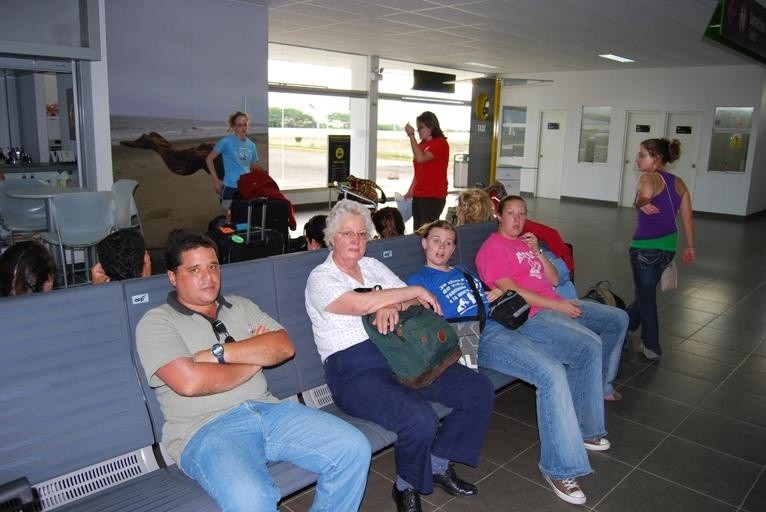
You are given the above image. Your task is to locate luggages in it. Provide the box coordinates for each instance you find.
[232,190,289,253]
[208,197,284,263]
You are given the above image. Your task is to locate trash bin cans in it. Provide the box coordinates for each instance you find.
[453,153,469,188]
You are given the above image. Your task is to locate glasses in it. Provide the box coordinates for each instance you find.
[213,318,235,343]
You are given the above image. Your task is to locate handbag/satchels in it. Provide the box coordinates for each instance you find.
[659,262,677,292]
[346,175,384,205]
[489,290,531,330]
[448,319,480,372]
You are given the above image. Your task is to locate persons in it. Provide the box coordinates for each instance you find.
[403,109,453,232]
[85,226,155,286]
[304,182,512,251]
[300,197,497,512]
[204,111,268,221]
[620,136,699,365]
[405,219,613,508]
[134,226,375,512]
[2,238,60,298]
[472,189,630,404]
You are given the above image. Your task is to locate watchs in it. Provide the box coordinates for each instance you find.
[532,248,545,257]
[210,344,228,363]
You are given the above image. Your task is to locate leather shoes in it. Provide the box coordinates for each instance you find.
[433,465,478,499]
[392,481,421,512]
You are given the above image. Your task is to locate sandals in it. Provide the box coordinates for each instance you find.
[606,392,621,402]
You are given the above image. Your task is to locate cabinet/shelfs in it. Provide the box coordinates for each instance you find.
[494,165,521,201]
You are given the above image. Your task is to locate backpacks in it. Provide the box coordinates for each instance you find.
[361,285,462,389]
[580,279,626,311]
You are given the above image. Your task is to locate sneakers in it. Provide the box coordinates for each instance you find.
[639,345,662,362]
[542,473,586,505]
[584,438,611,451]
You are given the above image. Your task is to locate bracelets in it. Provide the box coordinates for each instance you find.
[406,132,416,138]
[687,242,696,251]
[398,302,405,313]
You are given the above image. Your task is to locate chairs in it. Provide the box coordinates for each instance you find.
[1,172,146,287]
